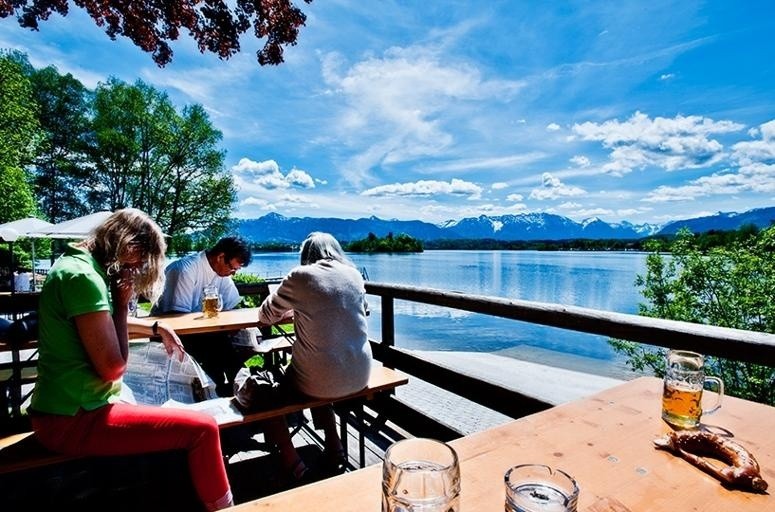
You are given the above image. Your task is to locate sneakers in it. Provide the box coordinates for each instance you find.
[315,446,346,471]
[262,457,312,491]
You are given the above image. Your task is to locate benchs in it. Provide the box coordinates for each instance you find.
[1,360,409,470]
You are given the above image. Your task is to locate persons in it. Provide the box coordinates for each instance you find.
[13,265,32,292]
[146,234,257,399]
[29,204,237,511]
[259,229,374,488]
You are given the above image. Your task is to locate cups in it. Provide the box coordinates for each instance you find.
[202,285,224,319]
[661,350,725,428]
[380,437,462,512]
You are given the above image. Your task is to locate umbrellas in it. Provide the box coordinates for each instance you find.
[0,214,57,293]
[24,211,116,241]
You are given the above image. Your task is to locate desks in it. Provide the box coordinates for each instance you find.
[204,374,774,510]
[127,308,296,340]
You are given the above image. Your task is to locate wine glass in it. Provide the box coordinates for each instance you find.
[128,294,139,317]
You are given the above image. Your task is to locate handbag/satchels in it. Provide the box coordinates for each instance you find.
[232,362,290,413]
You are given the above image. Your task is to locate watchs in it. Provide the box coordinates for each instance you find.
[150,320,159,336]
